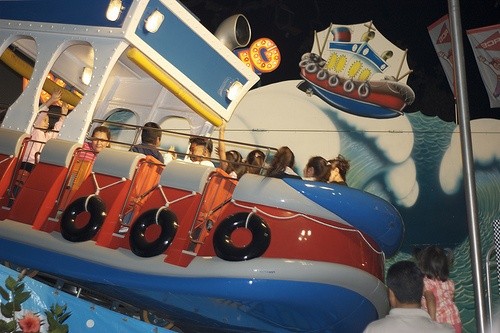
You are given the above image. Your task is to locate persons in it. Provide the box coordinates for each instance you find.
[415,243,463,333]
[7,87,70,206]
[362,261,456,333]
[165,135,350,187]
[129,122,164,167]
[87,125,112,155]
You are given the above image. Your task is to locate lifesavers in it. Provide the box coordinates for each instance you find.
[129,207,178,259]
[59,196,106,243]
[212,211,272,262]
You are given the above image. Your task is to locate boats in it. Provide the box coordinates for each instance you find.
[0,0,406,333]
